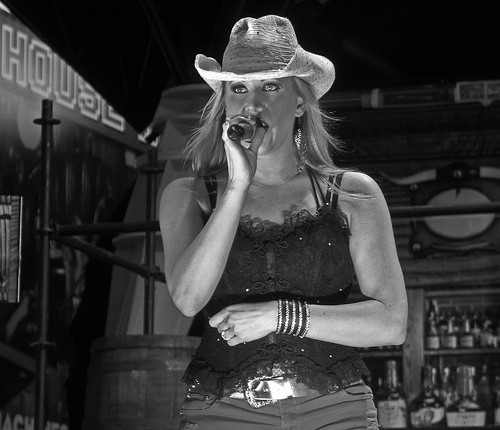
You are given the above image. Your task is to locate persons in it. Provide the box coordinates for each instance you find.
[159,14,408,430]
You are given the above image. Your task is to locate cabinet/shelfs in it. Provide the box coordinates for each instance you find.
[356,290,500,430]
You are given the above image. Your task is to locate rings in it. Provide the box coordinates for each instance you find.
[221,330,230,341]
[225,117,230,125]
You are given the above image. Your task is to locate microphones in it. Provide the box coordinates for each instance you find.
[226,116,262,142]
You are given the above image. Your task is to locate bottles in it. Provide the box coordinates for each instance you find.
[357,304,500,430]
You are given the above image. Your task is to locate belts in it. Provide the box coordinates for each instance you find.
[230,373,366,408]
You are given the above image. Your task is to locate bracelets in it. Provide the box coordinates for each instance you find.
[275,297,311,338]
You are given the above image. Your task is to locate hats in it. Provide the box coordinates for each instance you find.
[195,14,335,101]
